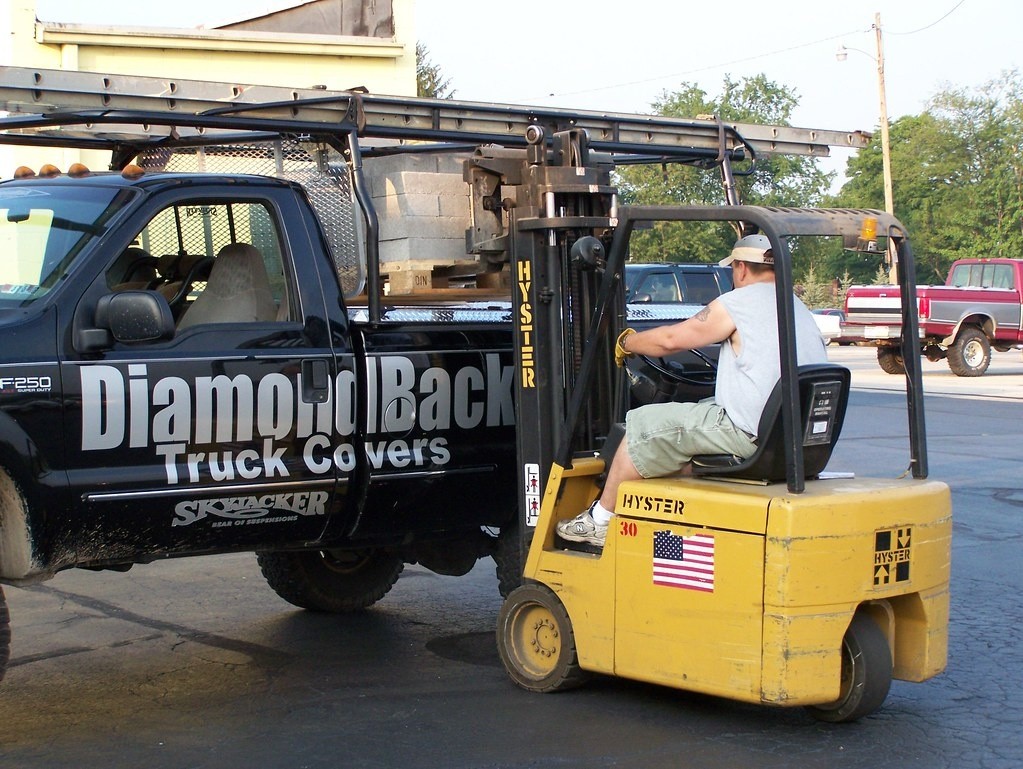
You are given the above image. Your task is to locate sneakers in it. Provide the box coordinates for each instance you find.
[555,499,608,546]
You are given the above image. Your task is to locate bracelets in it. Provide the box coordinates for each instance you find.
[622,334,628,346]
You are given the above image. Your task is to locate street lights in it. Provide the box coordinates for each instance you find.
[836,45,898,285]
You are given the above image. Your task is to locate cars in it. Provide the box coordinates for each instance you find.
[811,308,851,346]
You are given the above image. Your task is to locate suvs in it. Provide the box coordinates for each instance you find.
[625,265,734,333]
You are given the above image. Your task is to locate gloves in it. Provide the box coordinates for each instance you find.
[614,328,637,368]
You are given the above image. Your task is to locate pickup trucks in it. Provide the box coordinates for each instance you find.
[839,258,1023,376]
[0,66,873,680]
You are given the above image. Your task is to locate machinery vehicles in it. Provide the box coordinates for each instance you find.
[346,124,950,722]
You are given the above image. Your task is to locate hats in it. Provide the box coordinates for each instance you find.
[718,234,775,267]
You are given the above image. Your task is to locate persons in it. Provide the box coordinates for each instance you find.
[556,235,828,546]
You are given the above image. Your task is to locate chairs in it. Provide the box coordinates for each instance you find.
[105,246,156,298]
[174,243,278,334]
[689,363,850,486]
[653,284,678,303]
